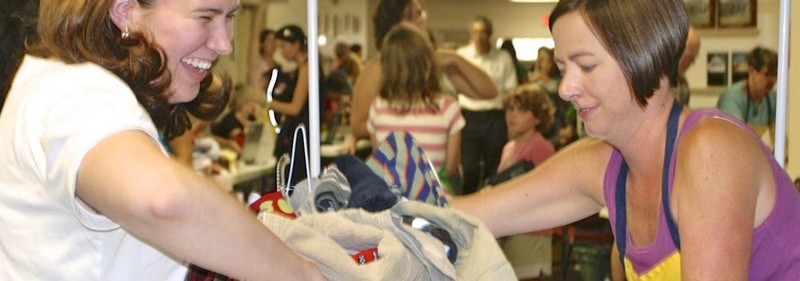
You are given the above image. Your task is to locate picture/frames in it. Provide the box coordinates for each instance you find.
[682,0,715,27]
[717,0,757,28]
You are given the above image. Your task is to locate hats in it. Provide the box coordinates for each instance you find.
[748,47,778,75]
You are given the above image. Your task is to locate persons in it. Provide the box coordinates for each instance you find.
[0,0,800,281]
[716,46,776,149]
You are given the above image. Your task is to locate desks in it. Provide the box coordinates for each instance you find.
[321,138,369,166]
[232,156,276,202]
